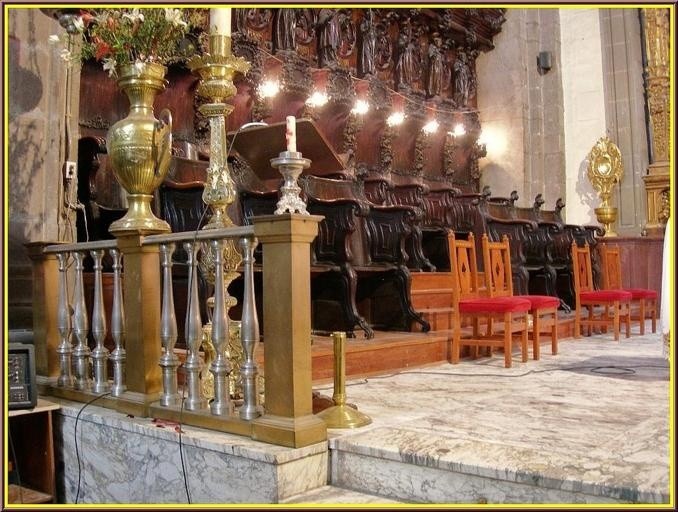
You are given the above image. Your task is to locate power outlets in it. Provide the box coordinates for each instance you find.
[66,161,77,178]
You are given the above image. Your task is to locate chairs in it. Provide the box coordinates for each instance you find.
[571,241,633,341]
[600,242,656,334]
[448,229,531,368]
[481,232,559,360]
[394,188,605,313]
[160,151,438,340]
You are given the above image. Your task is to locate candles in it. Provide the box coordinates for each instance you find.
[210,7,233,36]
[285,116,298,152]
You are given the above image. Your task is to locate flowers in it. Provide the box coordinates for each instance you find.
[47,9,208,79]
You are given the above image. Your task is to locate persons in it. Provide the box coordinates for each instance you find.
[394,24,416,91]
[452,52,472,106]
[427,38,444,99]
[357,11,377,78]
[315,8,342,69]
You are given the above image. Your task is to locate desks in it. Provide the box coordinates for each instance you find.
[8,397,62,503]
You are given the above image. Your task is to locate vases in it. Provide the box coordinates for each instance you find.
[106,63,173,237]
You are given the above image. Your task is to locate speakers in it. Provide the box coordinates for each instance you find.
[539,51,552,70]
